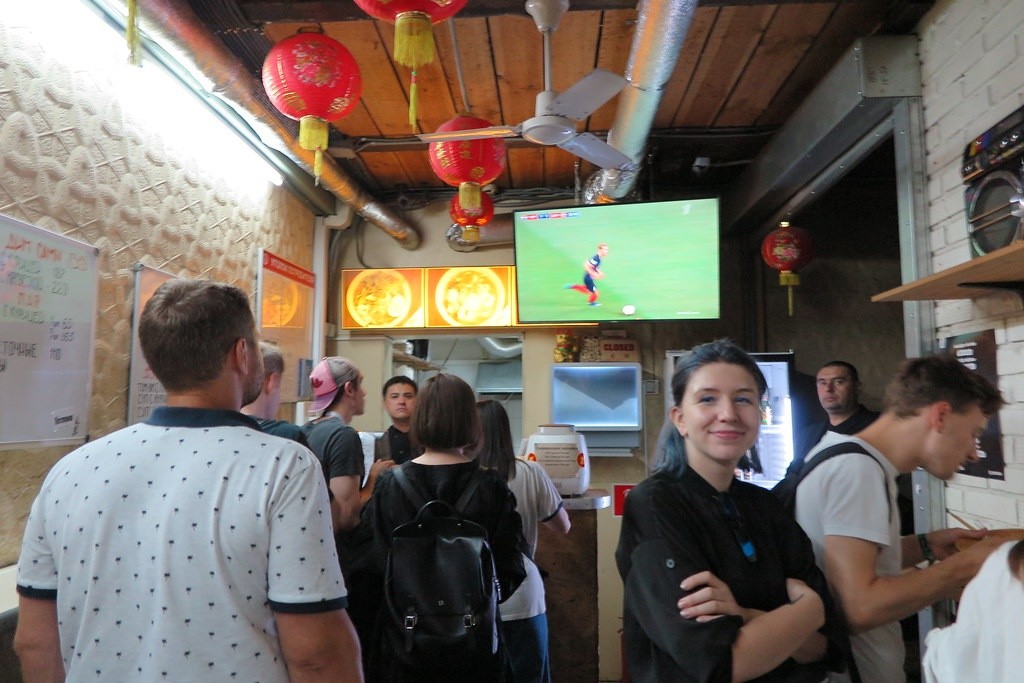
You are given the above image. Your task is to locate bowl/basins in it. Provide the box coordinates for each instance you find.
[262,274,299,327]
[435,267,505,327]
[345,268,412,328]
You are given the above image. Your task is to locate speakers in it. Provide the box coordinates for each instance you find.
[964,154,1024,257]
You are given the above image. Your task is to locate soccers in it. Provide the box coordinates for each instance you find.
[622,304,636,316]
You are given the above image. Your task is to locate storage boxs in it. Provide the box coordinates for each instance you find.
[600,339,643,362]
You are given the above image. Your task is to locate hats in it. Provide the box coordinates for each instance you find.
[309,357,359,414]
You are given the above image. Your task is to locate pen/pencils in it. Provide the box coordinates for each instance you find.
[945,507,979,531]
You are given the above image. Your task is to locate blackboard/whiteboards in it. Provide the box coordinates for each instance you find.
[126,263,180,426]
[0,213,101,451]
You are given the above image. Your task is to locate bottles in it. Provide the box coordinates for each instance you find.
[760,394,773,426]
[735,447,754,481]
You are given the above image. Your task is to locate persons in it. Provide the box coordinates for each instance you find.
[770,352,1013,683]
[242,340,341,535]
[462,400,572,683]
[300,357,395,530]
[785,361,882,477]
[614,337,848,683]
[374,375,427,468]
[922,538,1024,683]
[338,373,526,683]
[562,242,608,307]
[14,279,364,682]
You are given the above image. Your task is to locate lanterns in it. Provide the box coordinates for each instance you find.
[262,27,362,177]
[761,221,817,317]
[355,0,469,134]
[429,112,507,208]
[449,191,494,243]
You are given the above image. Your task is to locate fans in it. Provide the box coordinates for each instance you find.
[414,0,634,170]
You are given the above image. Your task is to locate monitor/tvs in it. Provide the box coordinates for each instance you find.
[665,349,803,490]
[512,194,724,324]
[475,359,524,392]
[551,363,643,431]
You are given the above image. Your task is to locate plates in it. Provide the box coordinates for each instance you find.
[955,528,1024,552]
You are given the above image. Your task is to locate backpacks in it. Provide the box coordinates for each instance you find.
[379,463,500,673]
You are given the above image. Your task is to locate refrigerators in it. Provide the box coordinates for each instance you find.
[663,346,805,492]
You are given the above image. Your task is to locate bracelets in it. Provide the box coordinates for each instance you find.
[917,530,936,562]
